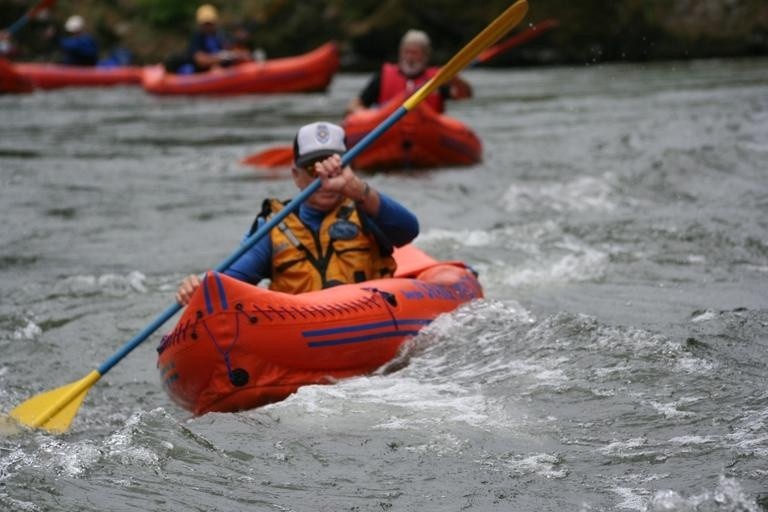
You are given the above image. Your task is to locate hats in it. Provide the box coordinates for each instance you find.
[293,120,349,166]
[196,4,220,27]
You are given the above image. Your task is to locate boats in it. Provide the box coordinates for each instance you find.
[341,87,481,175]
[157,245,484,425]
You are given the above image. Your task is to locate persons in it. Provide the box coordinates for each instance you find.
[341,26,474,116]
[56,12,99,66]
[172,118,419,309]
[184,3,253,71]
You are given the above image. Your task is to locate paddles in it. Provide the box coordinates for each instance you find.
[235,18,560,172]
[2,1,531,442]
